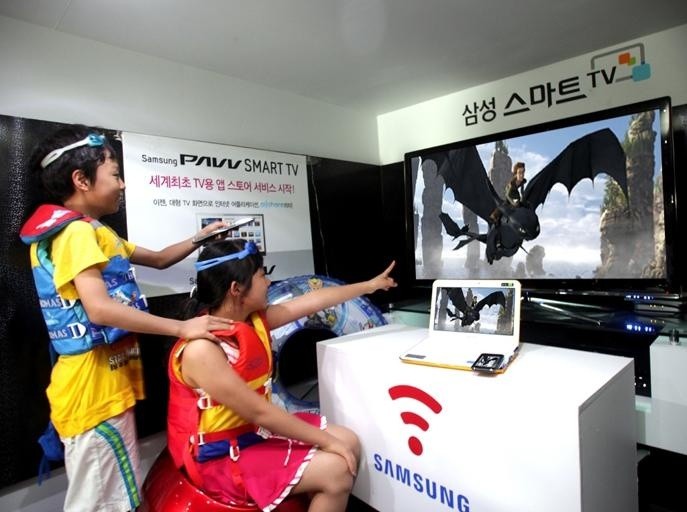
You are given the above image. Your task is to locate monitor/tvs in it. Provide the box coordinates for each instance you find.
[404,96,680,321]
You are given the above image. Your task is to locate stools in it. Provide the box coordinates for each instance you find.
[135,446,297,511]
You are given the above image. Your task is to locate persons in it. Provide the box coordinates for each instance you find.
[490,163,526,220]
[18,124,235,512]
[166,239,398,512]
[471,296,477,310]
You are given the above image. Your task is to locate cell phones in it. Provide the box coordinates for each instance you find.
[473,352,503,373]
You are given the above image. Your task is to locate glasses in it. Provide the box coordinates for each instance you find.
[195,241,257,272]
[41,134,106,167]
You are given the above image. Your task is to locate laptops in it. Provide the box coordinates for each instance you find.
[401,280,522,375]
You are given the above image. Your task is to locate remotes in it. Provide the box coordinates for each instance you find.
[192,212,254,243]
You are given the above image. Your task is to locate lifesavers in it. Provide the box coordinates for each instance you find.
[267,274,388,413]
